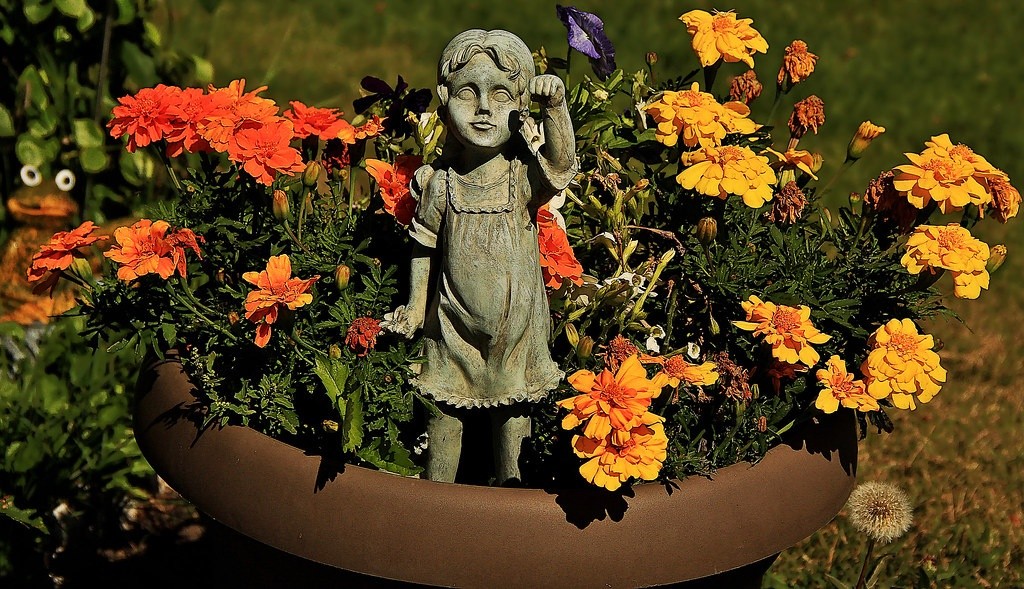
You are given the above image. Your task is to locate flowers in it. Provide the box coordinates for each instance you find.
[24,2,1024,492]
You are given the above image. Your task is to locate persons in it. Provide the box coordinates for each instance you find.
[390,25,578,489]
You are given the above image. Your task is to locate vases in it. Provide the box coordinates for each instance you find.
[131,348,857,589]
